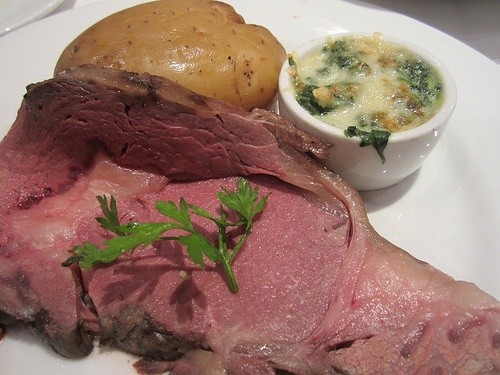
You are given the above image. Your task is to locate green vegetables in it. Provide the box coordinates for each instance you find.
[66,177,270,296]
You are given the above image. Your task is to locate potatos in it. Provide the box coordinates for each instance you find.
[54,1,288,112]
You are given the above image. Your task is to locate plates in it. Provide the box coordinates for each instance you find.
[1,0,65,36]
[1,0,500,374]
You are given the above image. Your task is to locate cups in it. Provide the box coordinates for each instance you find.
[278,33,457,191]
